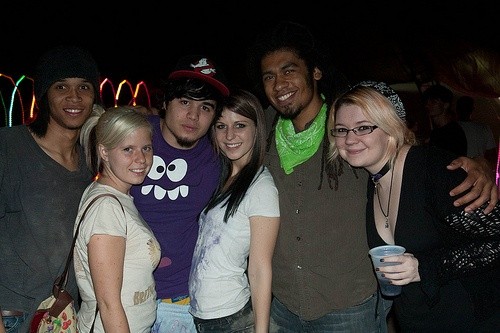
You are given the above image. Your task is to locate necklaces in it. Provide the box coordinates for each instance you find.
[377,144,399,228]
[371,159,392,194]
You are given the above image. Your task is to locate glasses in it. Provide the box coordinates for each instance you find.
[330,125,378,137]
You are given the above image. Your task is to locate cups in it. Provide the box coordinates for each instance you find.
[369,245,405,296]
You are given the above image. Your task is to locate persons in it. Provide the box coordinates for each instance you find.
[188,89,281,333]
[423,86,468,157]
[327,80,500,333]
[455,95,498,164]
[124,55,230,333]
[258,41,499,333]
[72,104,162,333]
[0,46,101,333]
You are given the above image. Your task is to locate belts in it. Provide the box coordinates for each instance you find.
[2,310,22,316]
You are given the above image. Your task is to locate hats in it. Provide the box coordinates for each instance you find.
[42,50,99,97]
[168,57,229,97]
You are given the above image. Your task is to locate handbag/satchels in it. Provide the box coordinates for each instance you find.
[30,288,76,333]
